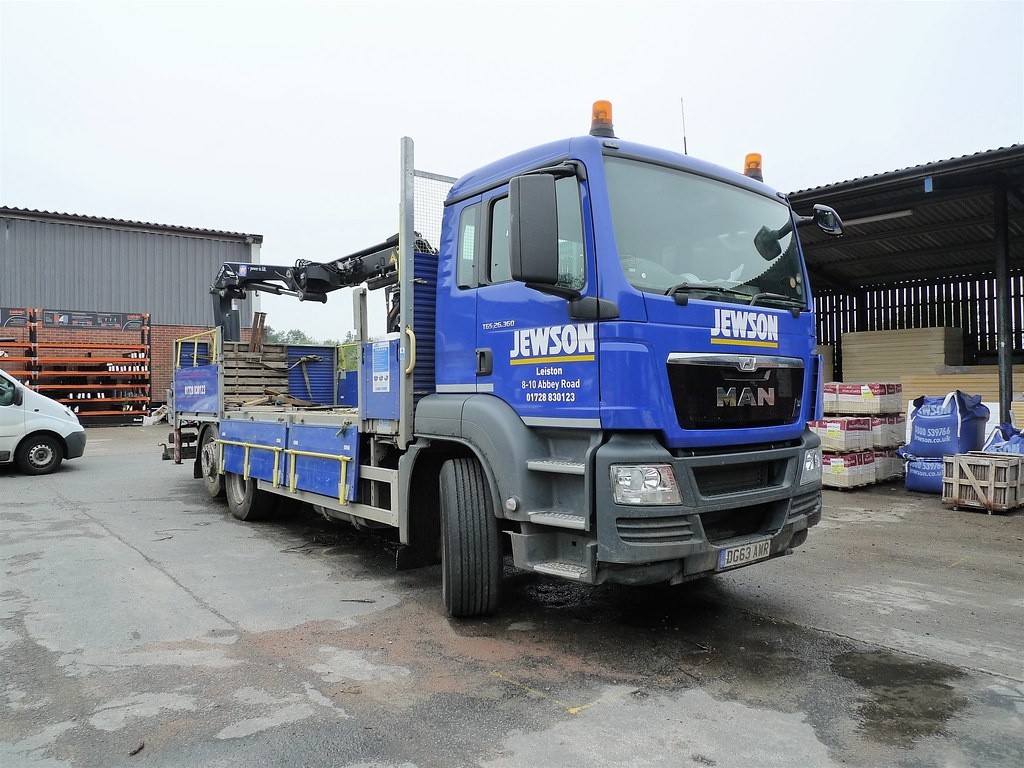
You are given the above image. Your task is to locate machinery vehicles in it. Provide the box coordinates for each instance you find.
[206,229,440,341]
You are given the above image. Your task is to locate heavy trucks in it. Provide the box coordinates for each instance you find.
[158,100,847,622]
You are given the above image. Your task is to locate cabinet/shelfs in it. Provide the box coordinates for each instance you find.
[0,307,153,426]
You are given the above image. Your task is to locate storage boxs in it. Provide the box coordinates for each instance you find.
[805,380,906,493]
[941,450,1024,514]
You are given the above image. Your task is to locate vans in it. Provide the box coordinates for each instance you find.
[0,369,87,478]
